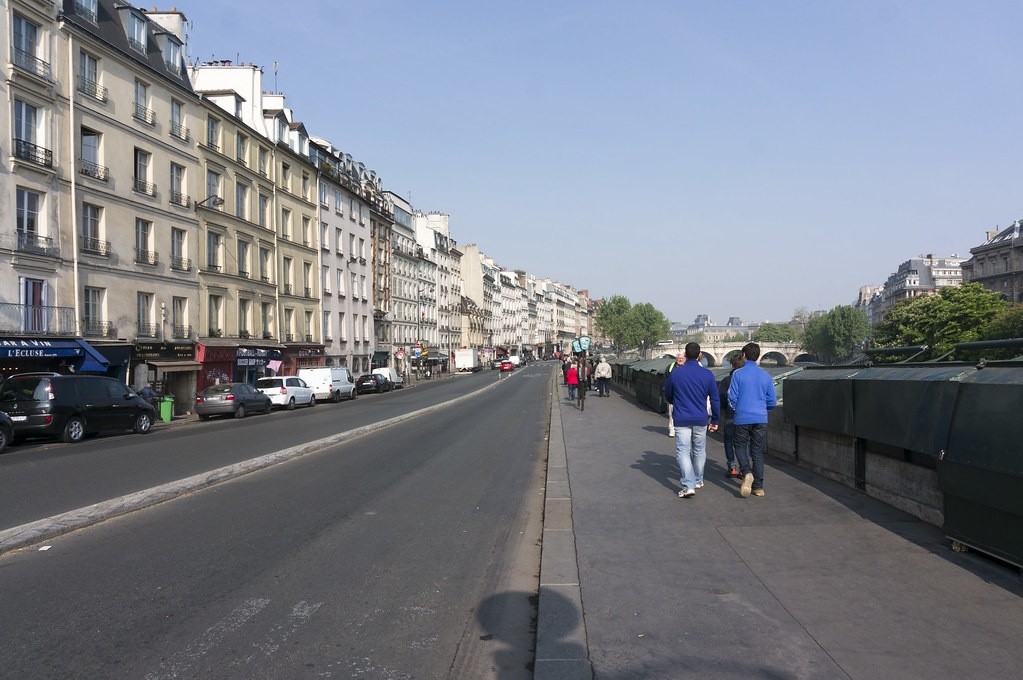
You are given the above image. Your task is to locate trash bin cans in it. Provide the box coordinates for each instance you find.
[159,396,174,423]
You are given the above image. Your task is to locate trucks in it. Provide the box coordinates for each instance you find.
[454,349,482,374]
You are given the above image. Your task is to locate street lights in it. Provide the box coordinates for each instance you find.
[418,287,435,339]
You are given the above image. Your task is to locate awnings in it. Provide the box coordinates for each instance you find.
[147,360,203,372]
[525,346,532,352]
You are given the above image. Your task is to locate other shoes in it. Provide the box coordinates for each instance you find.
[726,465,745,479]
[668,430,676,437]
[740,472,765,497]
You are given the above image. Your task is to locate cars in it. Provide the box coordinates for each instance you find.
[491,360,502,370]
[520,353,556,365]
[195,383,273,422]
[254,376,316,411]
[356,374,392,394]
[0,372,157,454]
[500,361,515,371]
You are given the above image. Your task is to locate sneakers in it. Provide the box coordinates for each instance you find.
[678,481,704,497]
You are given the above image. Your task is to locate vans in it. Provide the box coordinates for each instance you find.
[298,368,358,403]
[372,368,406,390]
[509,355,521,367]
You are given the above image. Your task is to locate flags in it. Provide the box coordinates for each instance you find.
[266,360,282,373]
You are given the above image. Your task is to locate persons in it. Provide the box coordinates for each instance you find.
[662,342,777,499]
[562,353,613,412]
[135,383,164,421]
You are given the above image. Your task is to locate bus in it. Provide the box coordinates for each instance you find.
[411,356,448,374]
[657,340,673,345]
